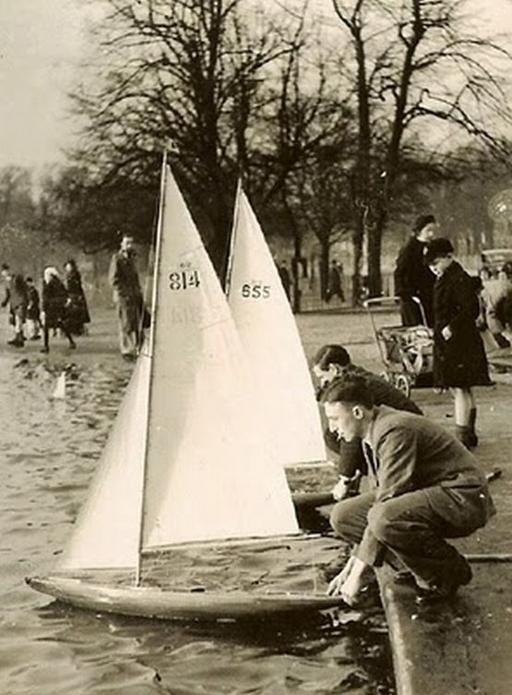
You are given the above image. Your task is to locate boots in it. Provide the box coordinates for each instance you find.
[457,426,469,448]
[469,408,478,447]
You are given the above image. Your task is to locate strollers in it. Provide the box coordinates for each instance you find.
[350,284,451,404]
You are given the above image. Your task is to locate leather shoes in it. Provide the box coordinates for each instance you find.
[415,569,473,605]
[392,569,413,584]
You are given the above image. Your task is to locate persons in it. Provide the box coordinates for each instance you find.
[38,262,80,353]
[322,259,347,308]
[279,259,291,304]
[392,212,437,328]
[24,276,44,343]
[318,376,498,614]
[474,280,512,378]
[62,257,92,338]
[419,236,493,452]
[478,261,512,289]
[1,263,31,349]
[310,342,425,505]
[355,276,369,308]
[107,228,153,364]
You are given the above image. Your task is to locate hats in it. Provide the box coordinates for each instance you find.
[415,214,436,228]
[422,238,455,265]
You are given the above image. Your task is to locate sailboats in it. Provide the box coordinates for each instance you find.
[22,139,354,635]
[213,178,363,516]
